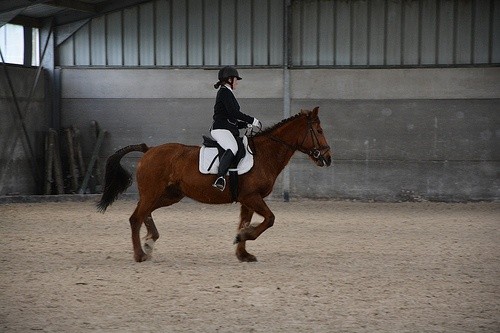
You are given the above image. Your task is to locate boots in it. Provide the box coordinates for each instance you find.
[214,149,234,189]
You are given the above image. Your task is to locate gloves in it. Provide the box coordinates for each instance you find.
[246,123,252,130]
[252,118,260,127]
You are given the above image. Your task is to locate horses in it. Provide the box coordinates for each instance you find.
[95,106,332,263]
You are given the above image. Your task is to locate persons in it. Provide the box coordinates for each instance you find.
[211,65,262,189]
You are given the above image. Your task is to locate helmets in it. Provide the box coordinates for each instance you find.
[218,66,243,82]
